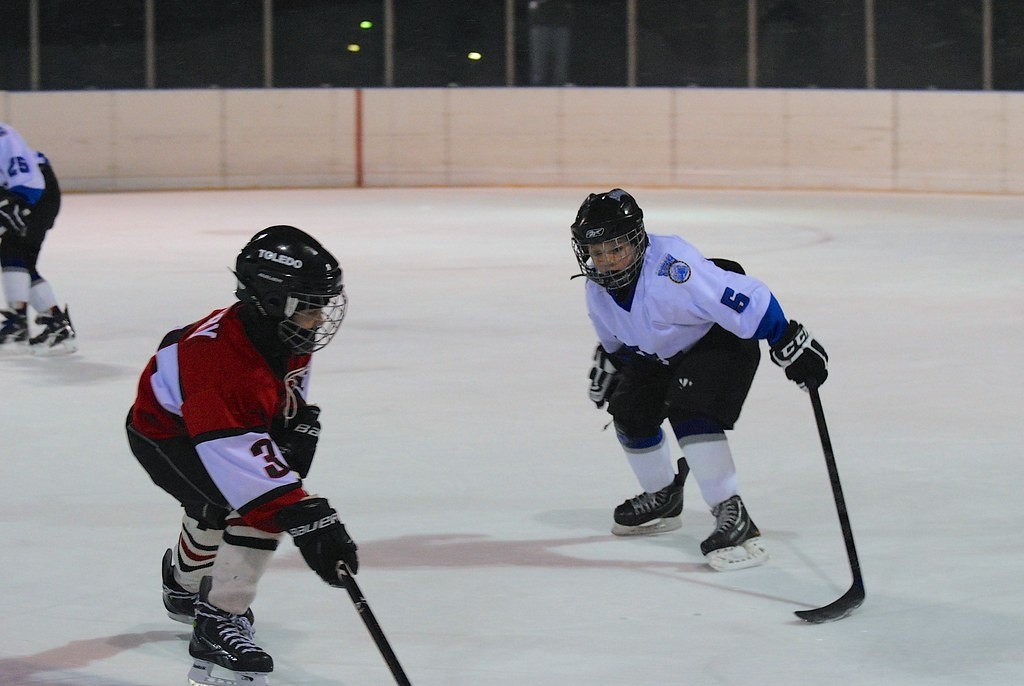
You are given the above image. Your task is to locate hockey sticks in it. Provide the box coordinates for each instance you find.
[335,560,412,686]
[792,375,867,625]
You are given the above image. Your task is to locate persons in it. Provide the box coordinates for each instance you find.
[125,225,359,673]
[0,122,76,349]
[570,188,828,556]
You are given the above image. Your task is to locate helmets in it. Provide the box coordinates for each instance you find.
[569,188,649,291]
[227,226,348,356]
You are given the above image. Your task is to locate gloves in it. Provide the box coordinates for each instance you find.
[589,348,622,409]
[278,405,320,478]
[278,498,359,588]
[769,320,828,392]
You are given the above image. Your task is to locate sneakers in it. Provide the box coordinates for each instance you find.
[162,549,256,635]
[611,457,690,537]
[188,575,275,686]
[0,303,31,354]
[700,496,767,573]
[29,305,78,357]
